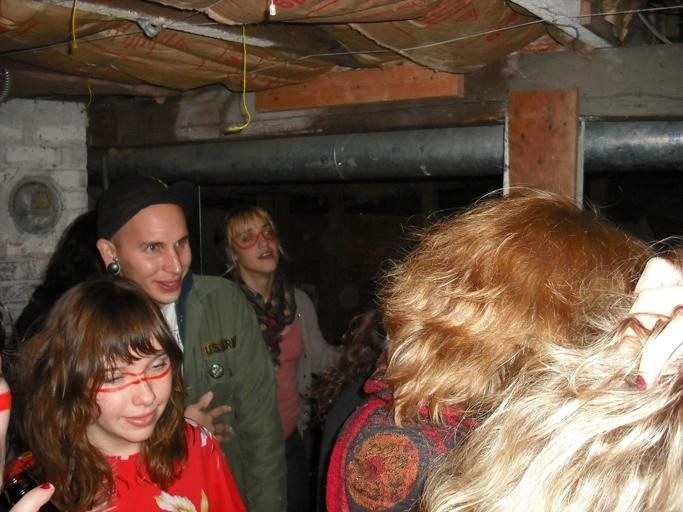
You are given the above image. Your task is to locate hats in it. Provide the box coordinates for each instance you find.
[95,172,195,239]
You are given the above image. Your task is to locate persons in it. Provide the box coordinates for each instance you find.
[221,202,345,512]
[307,311,384,512]
[2,277,249,512]
[416,249,683,512]
[323,187,659,512]
[88,174,290,512]
[0,377,56,512]
